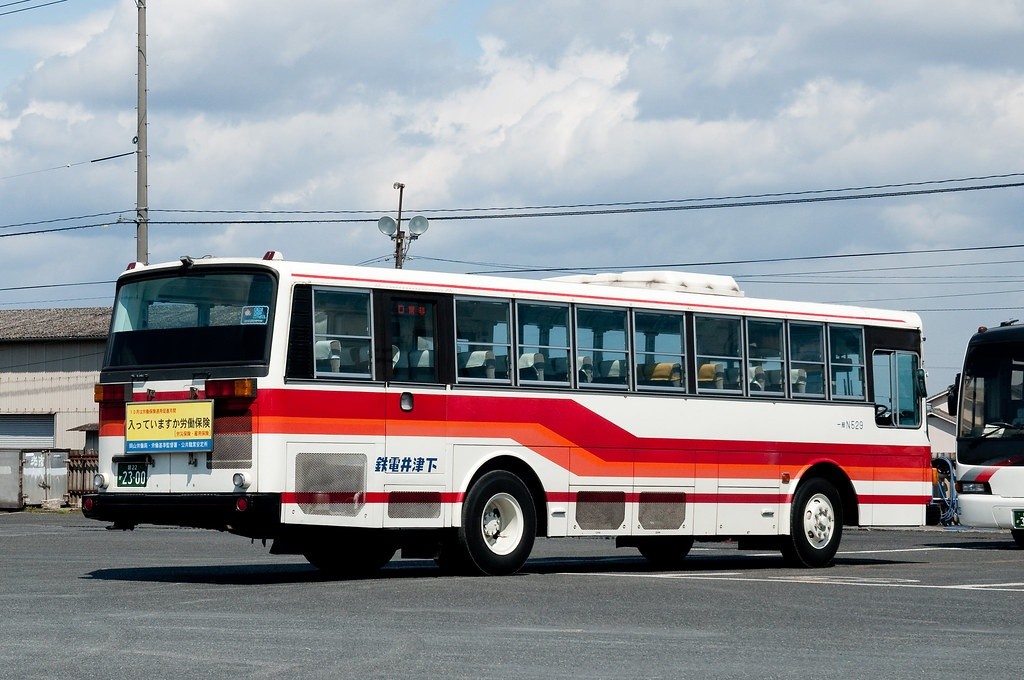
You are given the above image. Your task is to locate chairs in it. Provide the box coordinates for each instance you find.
[313,339,807,399]
[1012,408,1024,426]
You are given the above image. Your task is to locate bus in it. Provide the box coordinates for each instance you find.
[75,253,932,580]
[945,322,1024,546]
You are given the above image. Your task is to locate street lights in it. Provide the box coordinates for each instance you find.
[378,182,429,270]
